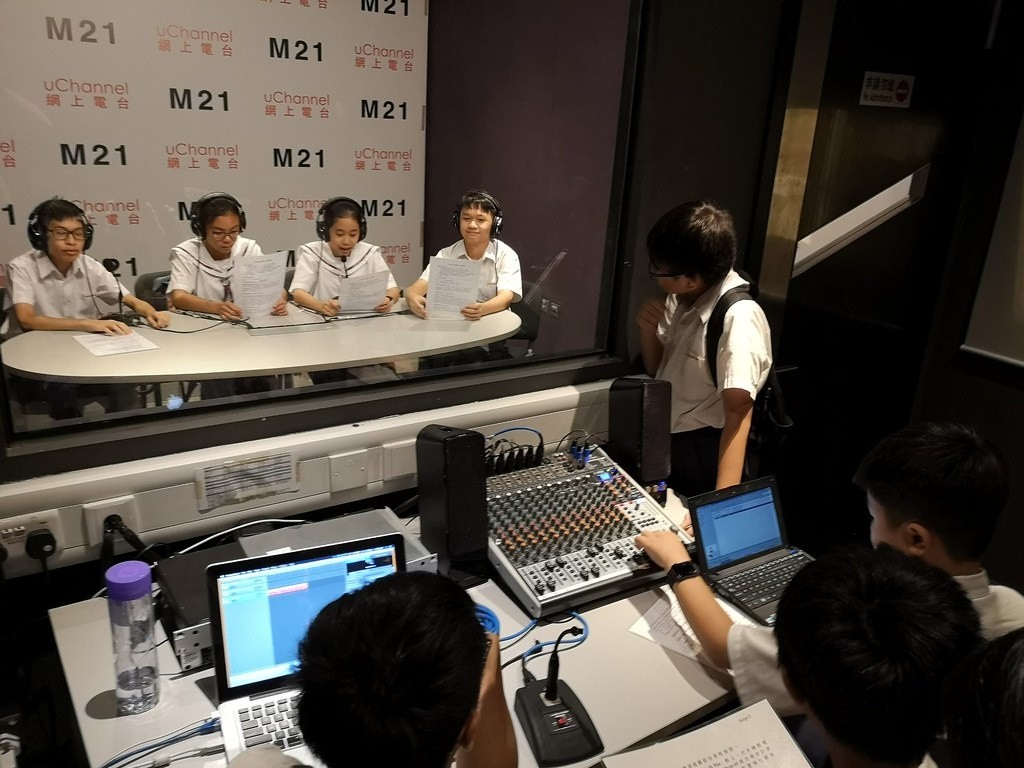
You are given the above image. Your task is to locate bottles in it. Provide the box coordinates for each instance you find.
[104,560,160,715]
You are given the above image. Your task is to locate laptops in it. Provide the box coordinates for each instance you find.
[204,529,407,768]
[686,474,816,628]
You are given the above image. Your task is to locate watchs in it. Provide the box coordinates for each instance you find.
[666,560,700,592]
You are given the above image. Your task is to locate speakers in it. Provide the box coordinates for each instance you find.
[413,425,488,589]
[607,378,671,507]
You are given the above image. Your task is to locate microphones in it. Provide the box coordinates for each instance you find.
[341,255,348,279]
[513,627,605,768]
[97,258,140,328]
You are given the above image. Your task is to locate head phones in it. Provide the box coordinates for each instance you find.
[453,193,503,238]
[28,201,93,251]
[316,196,367,245]
[189,192,246,238]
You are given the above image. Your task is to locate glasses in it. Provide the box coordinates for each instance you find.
[47,228,89,240]
[646,261,686,280]
[203,226,244,239]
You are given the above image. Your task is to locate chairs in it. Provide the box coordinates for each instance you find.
[135,270,172,311]
[501,280,542,358]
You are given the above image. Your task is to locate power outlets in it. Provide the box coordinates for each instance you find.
[83,495,141,547]
[0,509,64,563]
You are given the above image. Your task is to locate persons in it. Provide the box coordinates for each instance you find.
[774,540,988,768]
[635,196,772,537]
[289,197,399,316]
[7,199,171,336]
[636,422,1024,768]
[406,186,522,321]
[229,569,518,768]
[165,191,288,321]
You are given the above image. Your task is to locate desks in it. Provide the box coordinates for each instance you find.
[0,298,521,408]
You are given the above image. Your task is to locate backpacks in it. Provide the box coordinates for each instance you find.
[706,292,793,448]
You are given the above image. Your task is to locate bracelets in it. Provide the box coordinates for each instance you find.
[386,295,394,304]
